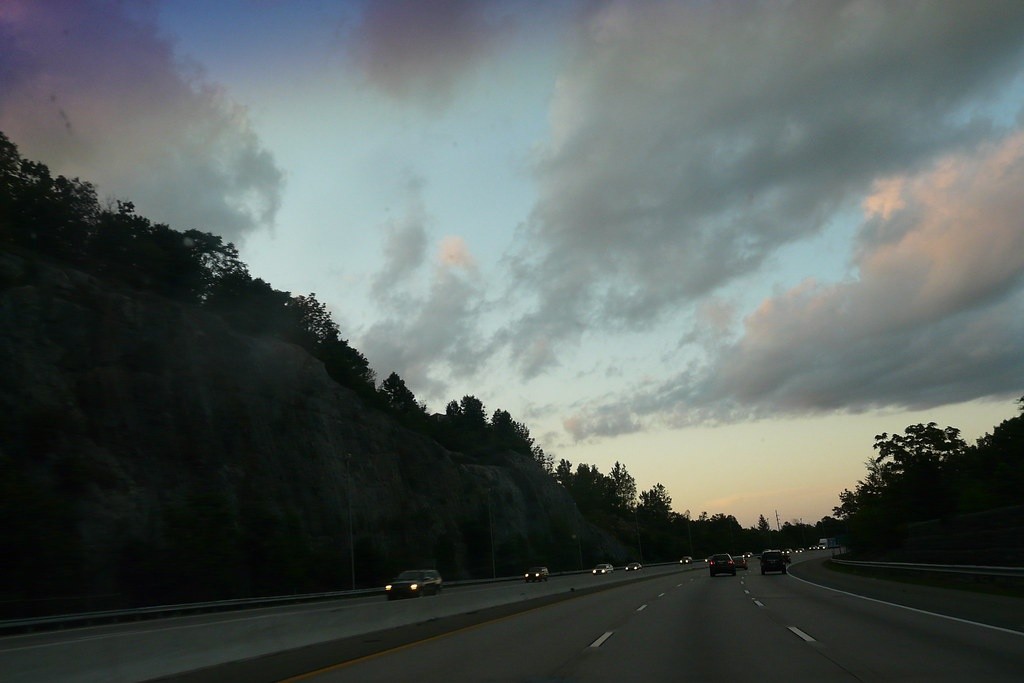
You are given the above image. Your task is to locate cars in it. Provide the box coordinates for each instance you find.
[625,563,643,571]
[705,552,753,577]
[680,555,693,564]
[760,550,787,575]
[593,563,614,575]
[782,544,825,563]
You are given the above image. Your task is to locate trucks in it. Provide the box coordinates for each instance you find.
[819,539,837,549]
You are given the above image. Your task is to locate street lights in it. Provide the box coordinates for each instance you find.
[342,453,353,590]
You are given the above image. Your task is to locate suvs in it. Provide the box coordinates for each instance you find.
[385,569,443,599]
[524,567,549,582]
